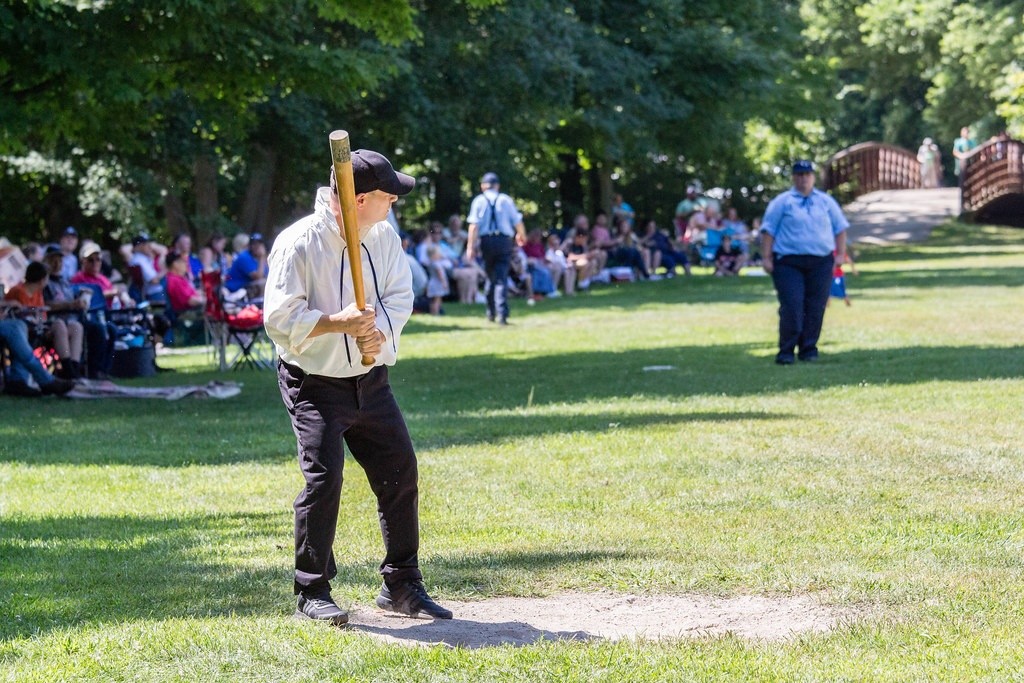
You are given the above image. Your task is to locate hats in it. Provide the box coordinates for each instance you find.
[165,251,181,267]
[79,241,101,258]
[330,149,415,196]
[481,172,500,183]
[45,246,64,257]
[64,226,79,236]
[250,231,265,242]
[791,160,815,172]
[132,235,147,247]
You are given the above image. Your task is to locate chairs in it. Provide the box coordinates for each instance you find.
[0,262,284,400]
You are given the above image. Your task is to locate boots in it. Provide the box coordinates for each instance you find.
[53,357,82,379]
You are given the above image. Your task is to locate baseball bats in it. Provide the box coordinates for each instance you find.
[328,128,377,368]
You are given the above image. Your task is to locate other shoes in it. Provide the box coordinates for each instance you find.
[488,314,507,324]
[39,379,73,396]
[798,355,819,361]
[2,379,38,398]
[544,290,562,298]
[88,370,111,380]
[527,297,536,306]
[776,353,794,364]
[528,295,542,301]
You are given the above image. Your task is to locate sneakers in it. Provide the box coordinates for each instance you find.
[376,579,453,619]
[295,587,348,626]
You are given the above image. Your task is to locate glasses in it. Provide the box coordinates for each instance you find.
[86,257,100,263]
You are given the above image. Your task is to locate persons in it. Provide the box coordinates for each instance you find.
[759,161,850,365]
[263,149,453,626]
[917,127,1008,189]
[466,173,526,325]
[387,184,762,317]
[0,227,274,398]
